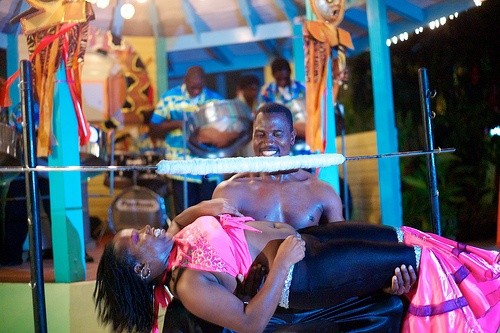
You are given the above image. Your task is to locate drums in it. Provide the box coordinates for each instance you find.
[107,185,166,235]
[137,151,164,180]
[0,120,22,166]
[103,150,137,189]
[21,122,108,184]
[284,99,306,137]
[186,100,253,159]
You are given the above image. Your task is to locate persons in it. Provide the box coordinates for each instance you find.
[162,102,417,333]
[91,198,500,333]
[261,57,306,125]
[151,67,226,219]
[229,75,258,108]
